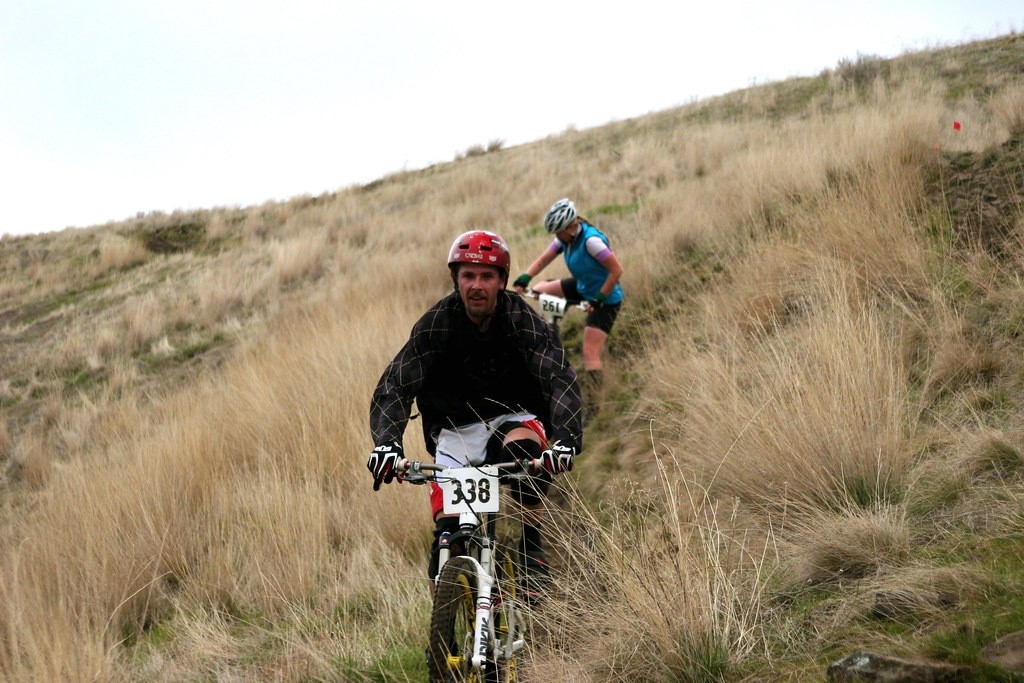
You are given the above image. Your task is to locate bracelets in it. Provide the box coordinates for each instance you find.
[519,274,531,284]
[597,292,608,303]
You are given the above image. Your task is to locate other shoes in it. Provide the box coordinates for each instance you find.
[520,550,547,604]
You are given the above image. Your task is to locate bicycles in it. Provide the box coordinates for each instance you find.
[379,449,574,683]
[515,278,606,340]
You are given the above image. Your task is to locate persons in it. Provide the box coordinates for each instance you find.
[367,229,584,614]
[511,198,625,428]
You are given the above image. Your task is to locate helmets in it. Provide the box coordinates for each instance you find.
[544,199,577,233]
[447,230,510,287]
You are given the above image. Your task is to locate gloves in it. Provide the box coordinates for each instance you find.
[367,441,406,492]
[541,438,575,476]
